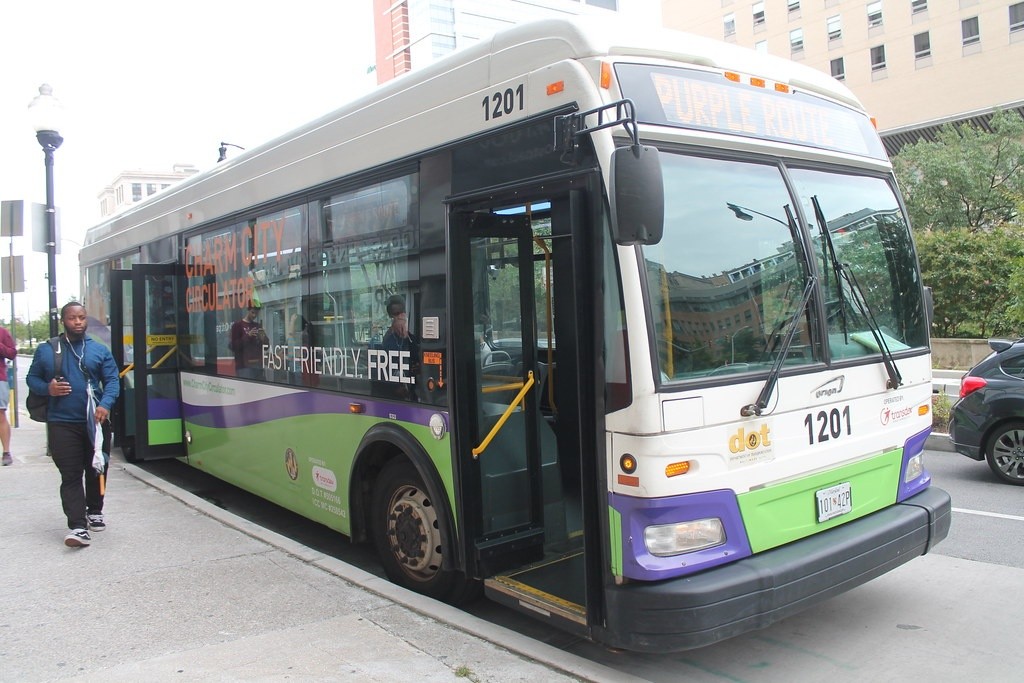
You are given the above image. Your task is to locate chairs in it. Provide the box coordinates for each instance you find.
[480,350,515,404]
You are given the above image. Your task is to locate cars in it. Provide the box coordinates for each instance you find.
[946,338,1024,484]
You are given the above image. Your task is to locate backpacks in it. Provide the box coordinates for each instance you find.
[26,337,63,422]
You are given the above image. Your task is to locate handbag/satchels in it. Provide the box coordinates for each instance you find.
[85,377,113,475]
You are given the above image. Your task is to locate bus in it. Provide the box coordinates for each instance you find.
[77,18,950,659]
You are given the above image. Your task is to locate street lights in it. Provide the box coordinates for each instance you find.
[366,32,453,76]
[28,84,62,344]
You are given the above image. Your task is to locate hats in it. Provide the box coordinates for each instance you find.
[384,295,406,318]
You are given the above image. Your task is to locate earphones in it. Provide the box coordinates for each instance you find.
[62,321,63,324]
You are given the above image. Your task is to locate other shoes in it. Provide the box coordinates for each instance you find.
[1,451,13,466]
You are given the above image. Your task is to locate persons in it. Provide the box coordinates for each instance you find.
[26,302,120,547]
[228,299,270,382]
[0,327,17,466]
[283,313,312,387]
[366,294,428,404]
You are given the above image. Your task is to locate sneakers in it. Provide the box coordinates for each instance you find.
[85,510,106,531]
[64,528,91,547]
